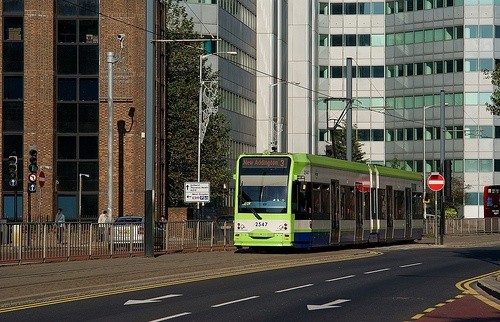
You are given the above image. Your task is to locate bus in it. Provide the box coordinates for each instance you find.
[233,150,424,247]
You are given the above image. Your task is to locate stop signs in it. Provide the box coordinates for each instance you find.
[357,174,375,193]
[426,173,445,191]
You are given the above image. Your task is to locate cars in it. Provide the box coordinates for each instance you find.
[108,216,167,245]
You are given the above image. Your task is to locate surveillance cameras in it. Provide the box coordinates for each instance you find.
[117,34,125,40]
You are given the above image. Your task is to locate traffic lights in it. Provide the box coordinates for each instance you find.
[9,155,18,187]
[29,150,38,173]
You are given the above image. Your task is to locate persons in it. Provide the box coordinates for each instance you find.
[158,216,168,227]
[56,208,66,244]
[98,210,108,242]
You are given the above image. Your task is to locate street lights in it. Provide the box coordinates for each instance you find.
[78,173,90,245]
[423,102,449,219]
[270,81,299,150]
[196,51,238,219]
